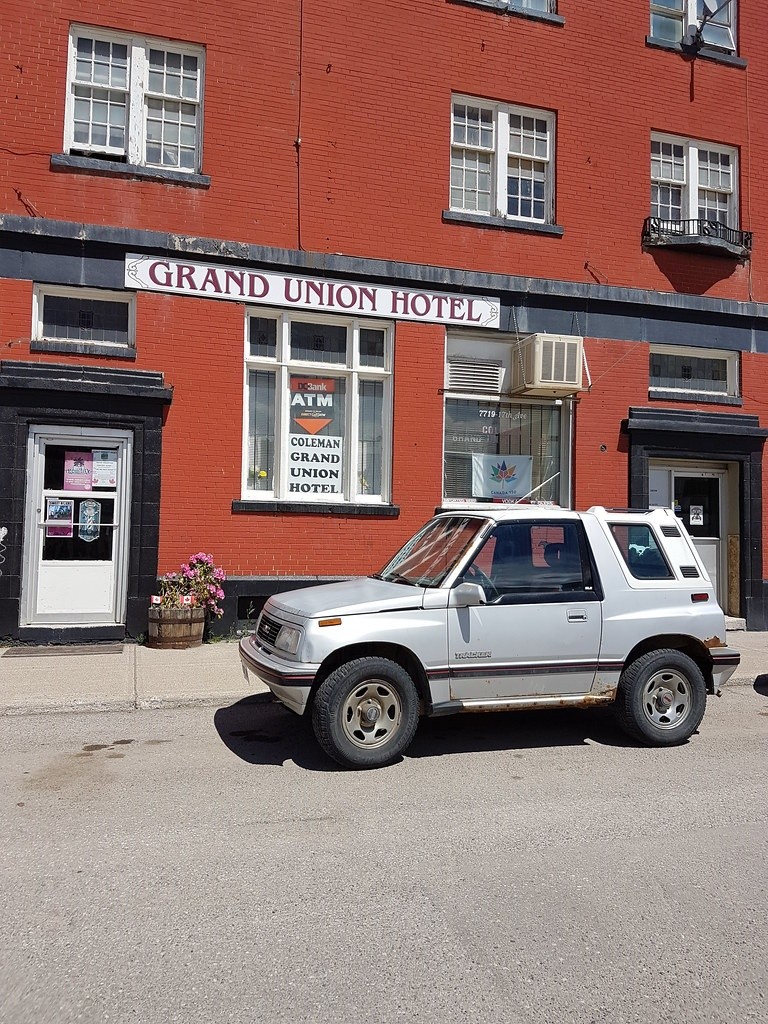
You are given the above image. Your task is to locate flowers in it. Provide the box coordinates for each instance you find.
[159,553,226,618]
[249,465,267,480]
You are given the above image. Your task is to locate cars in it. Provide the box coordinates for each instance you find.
[239,496,741,769]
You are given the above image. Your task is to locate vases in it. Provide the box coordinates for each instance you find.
[249,477,261,489]
[148,606,205,650]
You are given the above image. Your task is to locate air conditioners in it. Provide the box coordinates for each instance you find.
[512,333,583,396]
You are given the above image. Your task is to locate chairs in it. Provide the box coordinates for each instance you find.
[538,542,577,591]
[628,545,670,577]
[496,540,527,593]
